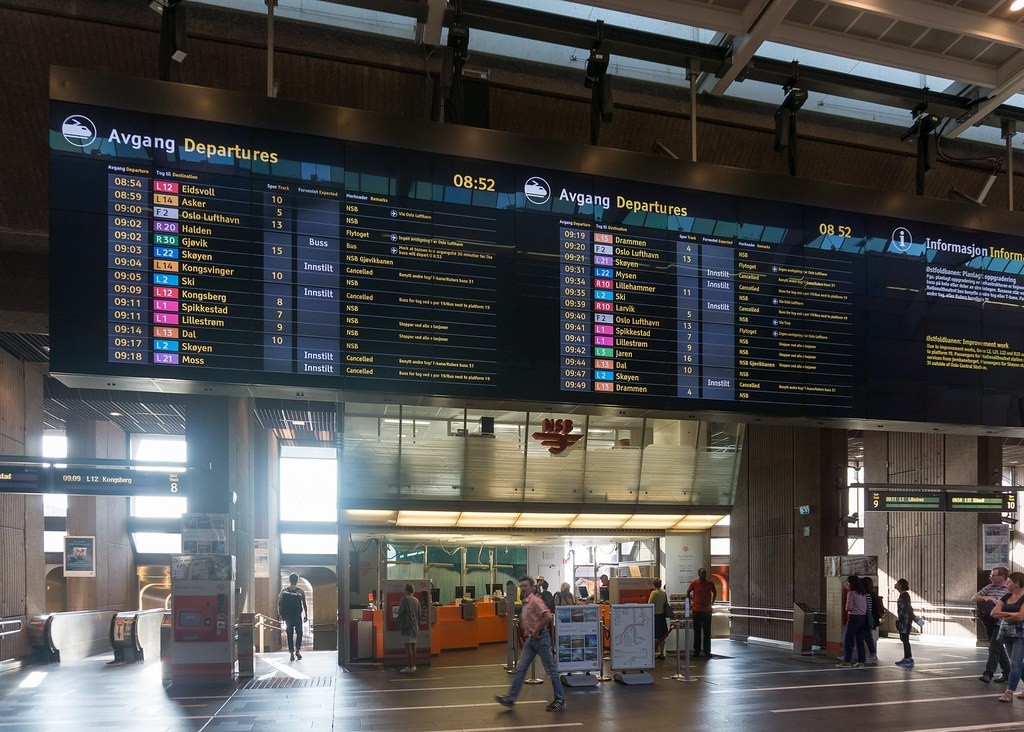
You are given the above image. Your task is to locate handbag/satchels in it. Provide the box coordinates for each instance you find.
[996,620,1016,642]
[395,597,415,630]
[664,602,674,618]
[873,595,885,618]
[867,611,874,629]
[895,619,905,631]
[977,601,999,625]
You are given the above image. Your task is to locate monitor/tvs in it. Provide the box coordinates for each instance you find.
[456,585,475,599]
[423,588,440,603]
[486,583,504,596]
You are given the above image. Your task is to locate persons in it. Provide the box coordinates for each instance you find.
[532,575,609,607]
[990,572,1024,703]
[894,579,918,666]
[835,575,881,668]
[971,567,1012,684]
[647,578,666,660]
[494,577,567,712]
[276,573,307,661]
[687,568,717,657]
[397,583,421,673]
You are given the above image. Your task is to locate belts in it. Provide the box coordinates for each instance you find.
[539,628,549,634]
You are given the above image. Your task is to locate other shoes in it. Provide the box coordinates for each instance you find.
[296,652,302,659]
[999,691,1014,702]
[400,666,416,673]
[979,674,991,684]
[852,662,864,667]
[865,657,878,663]
[894,659,914,667]
[993,672,1009,682]
[834,662,852,668]
[1017,690,1024,698]
[291,658,295,661]
[656,655,665,660]
[837,656,844,661]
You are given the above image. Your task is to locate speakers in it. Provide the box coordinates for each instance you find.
[480,416,495,435]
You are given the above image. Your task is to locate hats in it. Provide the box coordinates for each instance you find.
[535,575,544,580]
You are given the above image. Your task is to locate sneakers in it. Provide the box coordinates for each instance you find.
[546,700,566,712]
[495,695,513,710]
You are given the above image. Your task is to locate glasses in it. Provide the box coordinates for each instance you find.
[990,574,1003,579]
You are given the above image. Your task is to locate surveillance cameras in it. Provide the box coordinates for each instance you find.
[842,515,857,523]
[1002,517,1018,524]
[504,550,509,553]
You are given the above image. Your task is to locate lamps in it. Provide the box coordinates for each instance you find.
[465,564,490,570]
[425,562,454,572]
[384,560,412,566]
[493,564,514,569]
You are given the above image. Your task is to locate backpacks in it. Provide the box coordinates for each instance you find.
[281,587,302,620]
